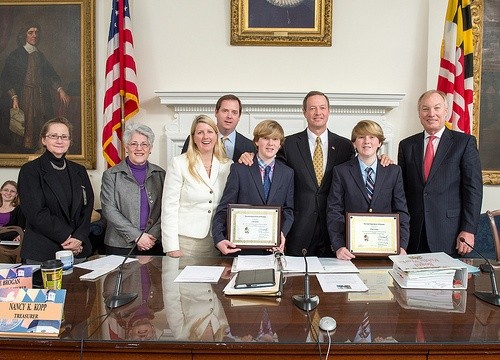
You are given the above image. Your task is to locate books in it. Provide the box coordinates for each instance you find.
[222,269,283,297]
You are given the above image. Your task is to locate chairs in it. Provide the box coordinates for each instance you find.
[486,210,500,264]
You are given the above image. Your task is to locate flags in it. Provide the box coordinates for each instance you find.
[437,0,474,135]
[101,0,140,169]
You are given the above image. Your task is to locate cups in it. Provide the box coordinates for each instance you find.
[41,260,64,290]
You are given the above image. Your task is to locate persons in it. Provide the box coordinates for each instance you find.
[0,181,20,227]
[161,256,231,342]
[327,119,411,260]
[180,94,256,163]
[212,120,294,258]
[222,257,476,343]
[62,268,96,338]
[103,255,164,340]
[0,205,26,264]
[398,89,484,257]
[17,116,93,265]
[161,115,234,257]
[100,122,165,256]
[237,91,395,258]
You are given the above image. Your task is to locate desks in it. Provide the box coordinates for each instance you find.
[0,254,500,360]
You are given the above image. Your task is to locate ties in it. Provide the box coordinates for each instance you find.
[365,167,376,203]
[424,136,436,184]
[310,308,321,342]
[312,137,324,187]
[261,306,271,332]
[416,319,425,342]
[263,166,272,204]
[360,309,370,338]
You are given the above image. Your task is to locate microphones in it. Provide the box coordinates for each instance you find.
[291,249,319,310]
[106,219,153,308]
[459,236,500,306]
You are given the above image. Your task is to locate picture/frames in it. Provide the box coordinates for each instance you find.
[226,203,282,249]
[0,0,97,171]
[230,0,333,48]
[344,211,401,257]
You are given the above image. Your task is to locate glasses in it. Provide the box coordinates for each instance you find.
[43,133,70,141]
[128,141,150,148]
